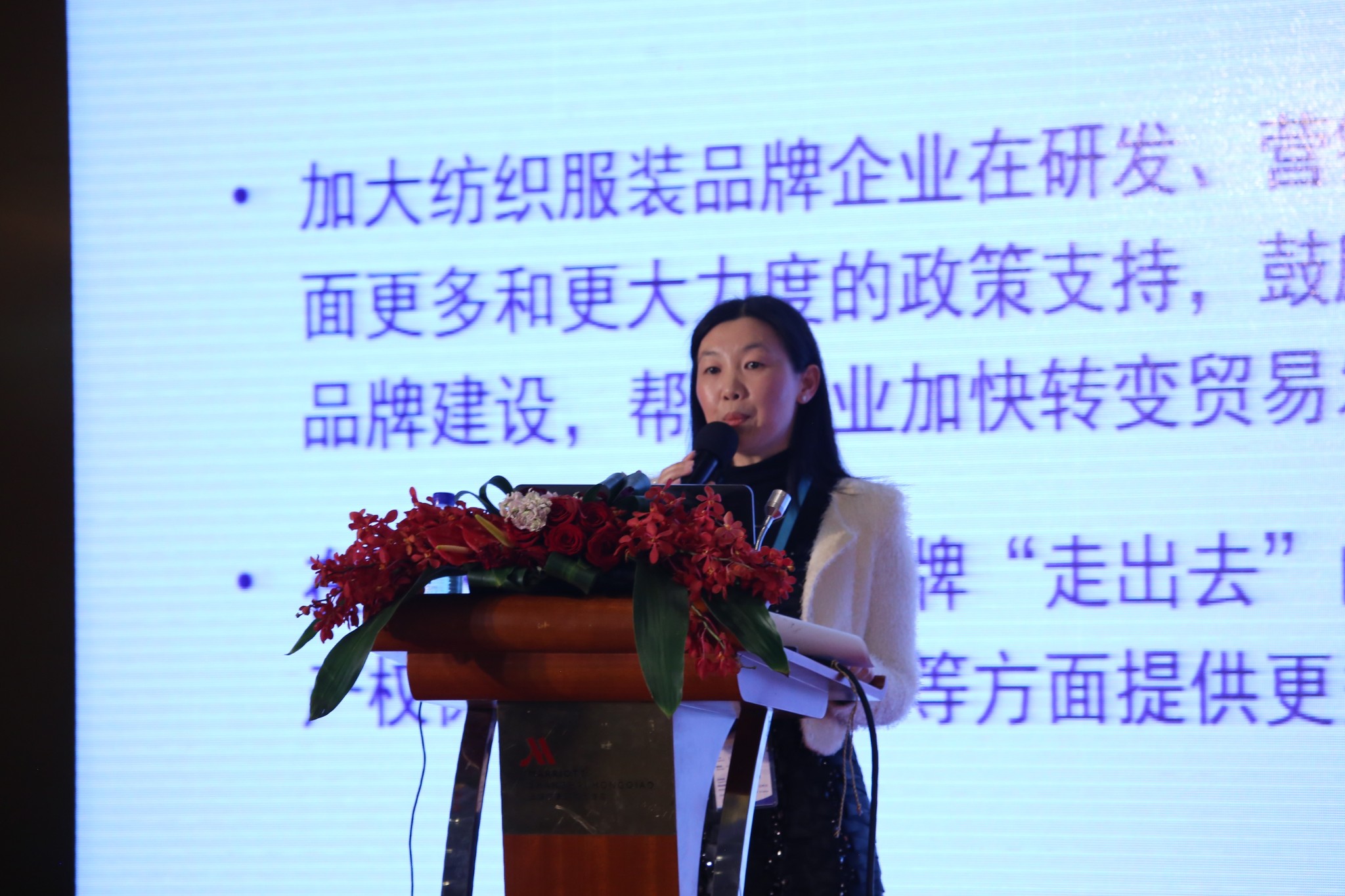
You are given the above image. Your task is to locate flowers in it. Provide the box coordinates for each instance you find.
[285,484,793,725]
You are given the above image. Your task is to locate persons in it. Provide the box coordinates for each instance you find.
[649,296,920,895]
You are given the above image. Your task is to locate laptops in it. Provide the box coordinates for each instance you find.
[515,483,757,552]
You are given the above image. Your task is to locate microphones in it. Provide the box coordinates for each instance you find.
[686,421,740,484]
[754,489,792,551]
